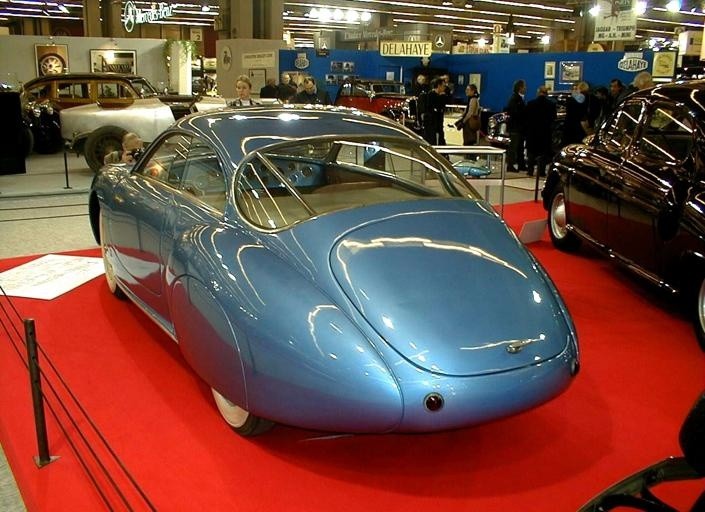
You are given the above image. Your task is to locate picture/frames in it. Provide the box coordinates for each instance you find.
[89,48,137,77]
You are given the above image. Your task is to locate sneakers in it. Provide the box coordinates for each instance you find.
[507,164,547,177]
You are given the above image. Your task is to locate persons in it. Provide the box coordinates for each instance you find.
[414,74,432,98]
[256,79,279,99]
[519,85,558,178]
[102,132,145,176]
[423,78,449,158]
[277,74,297,101]
[455,84,483,145]
[505,79,530,174]
[561,70,656,150]
[222,75,261,112]
[440,74,456,96]
[290,76,323,104]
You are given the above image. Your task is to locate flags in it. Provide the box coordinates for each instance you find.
[593,1,637,42]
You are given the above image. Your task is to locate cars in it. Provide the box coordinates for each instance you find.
[541,79,705,350]
[16,73,580,438]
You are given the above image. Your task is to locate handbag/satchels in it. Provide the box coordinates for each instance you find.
[465,115,482,132]
